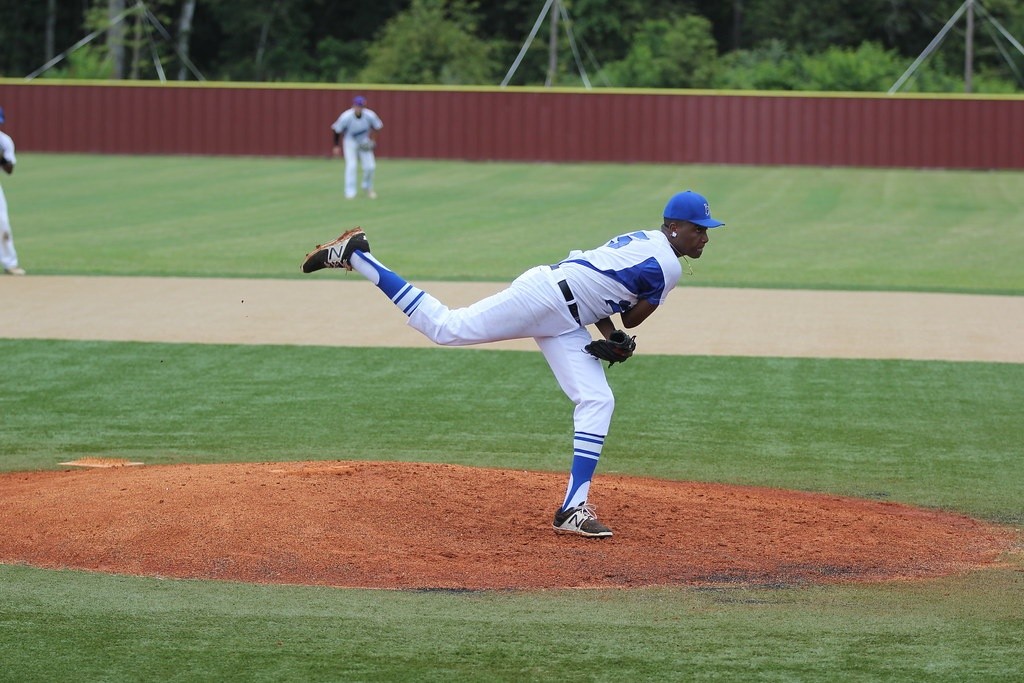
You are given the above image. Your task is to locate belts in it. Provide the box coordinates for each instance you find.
[551,263,582,328]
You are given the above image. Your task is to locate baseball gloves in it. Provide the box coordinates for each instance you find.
[584,329,637,368]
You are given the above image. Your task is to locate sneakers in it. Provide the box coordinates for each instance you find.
[552,507,615,534]
[7,267,28,275]
[301,226,369,274]
[366,192,377,198]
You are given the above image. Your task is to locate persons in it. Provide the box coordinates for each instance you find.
[329,96,384,199]
[0,107,27,277]
[297,189,728,540]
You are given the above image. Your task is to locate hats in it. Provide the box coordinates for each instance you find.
[354,96,368,107]
[663,191,724,228]
[0,106,5,124]
